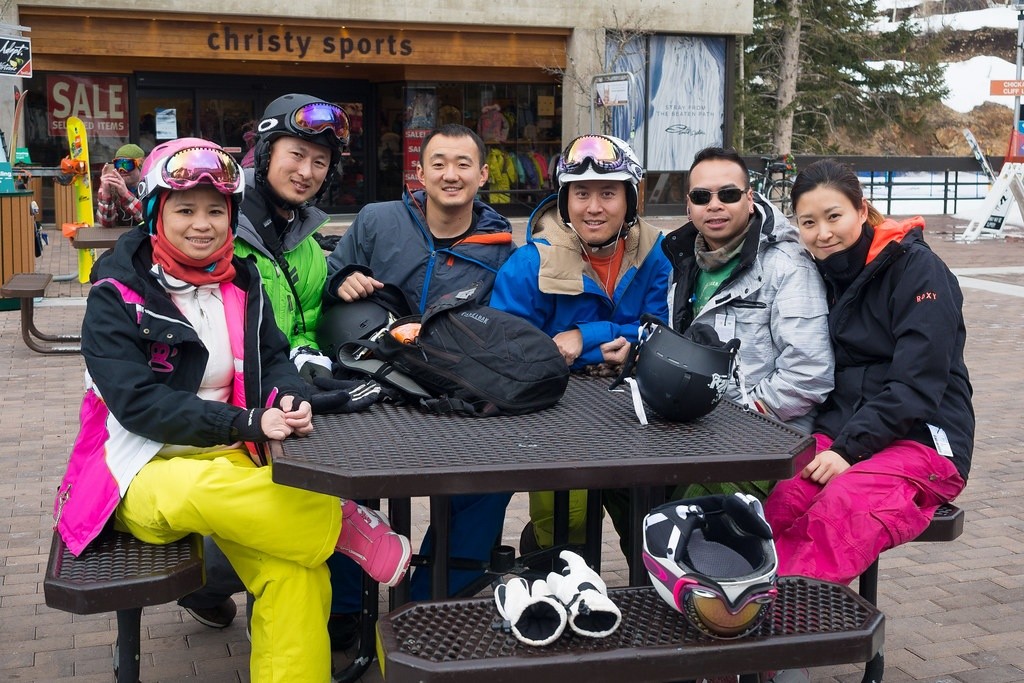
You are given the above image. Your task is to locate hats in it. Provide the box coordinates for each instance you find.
[114,143,145,171]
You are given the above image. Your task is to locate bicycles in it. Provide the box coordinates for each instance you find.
[748,157,794,219]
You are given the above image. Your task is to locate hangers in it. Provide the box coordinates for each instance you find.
[486,140,549,154]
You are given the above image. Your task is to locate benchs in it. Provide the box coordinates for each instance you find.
[0,274,82,353]
[44,499,965,683]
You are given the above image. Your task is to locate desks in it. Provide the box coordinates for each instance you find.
[266,370,816,612]
[74,227,136,248]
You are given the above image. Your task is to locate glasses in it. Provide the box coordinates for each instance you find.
[671,573,778,640]
[687,188,747,205]
[156,147,245,196]
[112,158,139,173]
[559,134,626,175]
[284,101,351,148]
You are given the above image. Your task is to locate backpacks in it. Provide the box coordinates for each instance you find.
[335,281,572,417]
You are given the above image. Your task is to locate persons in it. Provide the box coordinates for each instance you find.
[488,134,675,573]
[662,147,835,503]
[97,144,146,227]
[321,126,519,647]
[54,135,412,683]
[763,154,976,588]
[177,93,351,627]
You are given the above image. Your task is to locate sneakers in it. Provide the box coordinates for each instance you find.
[177,592,237,628]
[329,610,360,651]
[334,498,411,588]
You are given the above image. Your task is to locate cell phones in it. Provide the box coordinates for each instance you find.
[105,164,113,175]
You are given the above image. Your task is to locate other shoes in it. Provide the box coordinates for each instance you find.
[518,520,544,555]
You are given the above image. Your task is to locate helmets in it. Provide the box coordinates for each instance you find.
[318,300,389,361]
[254,94,344,198]
[140,138,239,235]
[633,314,740,422]
[644,493,778,616]
[558,136,642,227]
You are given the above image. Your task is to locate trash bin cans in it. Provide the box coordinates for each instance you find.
[0,190,35,312]
[53,177,76,230]
[14,162,43,222]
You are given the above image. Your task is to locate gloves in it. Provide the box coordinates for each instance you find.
[302,377,384,413]
[546,550,622,638]
[494,577,568,647]
[289,344,333,387]
[584,360,638,379]
